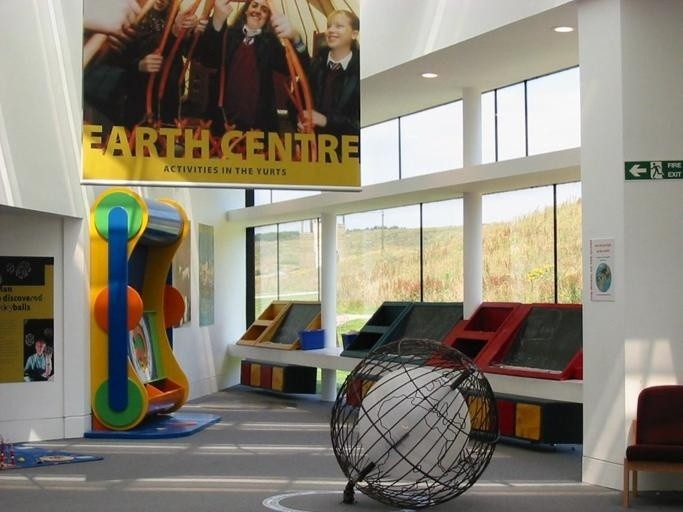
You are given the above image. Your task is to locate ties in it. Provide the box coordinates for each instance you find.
[244,37,251,46]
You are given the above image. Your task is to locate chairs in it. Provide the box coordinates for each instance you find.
[622,384,683,507]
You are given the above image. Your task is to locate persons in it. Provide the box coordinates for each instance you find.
[83,1,361,154]
[24,340,53,380]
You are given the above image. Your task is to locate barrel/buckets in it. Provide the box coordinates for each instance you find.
[297,329,325,351]
